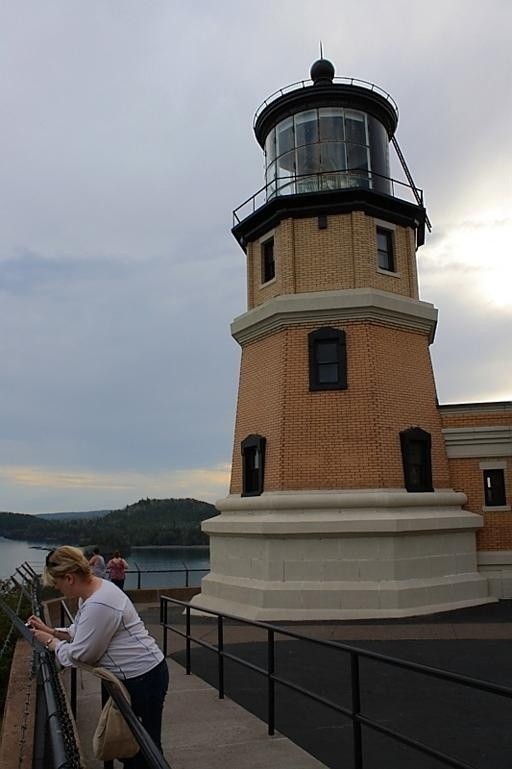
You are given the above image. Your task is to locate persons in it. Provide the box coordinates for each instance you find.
[27,545,169,769]
[90,547,105,578]
[108,550,128,589]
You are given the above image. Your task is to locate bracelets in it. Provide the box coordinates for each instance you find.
[45,636,53,650]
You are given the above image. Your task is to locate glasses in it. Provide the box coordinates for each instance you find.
[46,550,59,567]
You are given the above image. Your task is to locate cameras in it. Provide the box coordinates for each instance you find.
[25,619,35,631]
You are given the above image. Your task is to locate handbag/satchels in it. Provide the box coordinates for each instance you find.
[65,653,141,761]
